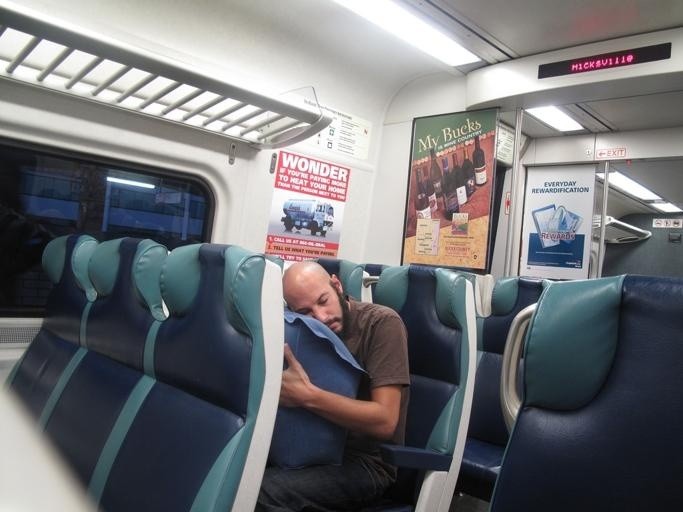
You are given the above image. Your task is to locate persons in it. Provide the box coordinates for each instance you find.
[251,258,413,511]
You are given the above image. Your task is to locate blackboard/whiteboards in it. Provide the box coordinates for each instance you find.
[280,199,334,235]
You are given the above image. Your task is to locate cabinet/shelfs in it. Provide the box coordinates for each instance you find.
[414,136,488,220]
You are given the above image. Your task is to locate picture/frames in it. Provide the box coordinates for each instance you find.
[398,107,501,274]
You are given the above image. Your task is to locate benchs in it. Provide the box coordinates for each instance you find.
[270,257,683,510]
[5,237,283,510]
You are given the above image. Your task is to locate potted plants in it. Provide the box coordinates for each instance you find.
[398,107,501,274]
